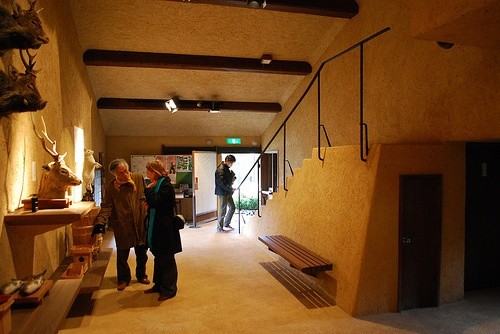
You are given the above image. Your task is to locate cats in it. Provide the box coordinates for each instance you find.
[82,148,102,196]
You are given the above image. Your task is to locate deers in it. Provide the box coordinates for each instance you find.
[30,113,83,199]
[0,0,50,57]
[0,49,48,120]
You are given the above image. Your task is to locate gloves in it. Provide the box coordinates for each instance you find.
[91,225,106,237]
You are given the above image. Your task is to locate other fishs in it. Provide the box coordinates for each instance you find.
[0,278,24,295]
[18,269,47,297]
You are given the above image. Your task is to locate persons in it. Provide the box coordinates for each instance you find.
[215,155,236,232]
[142,160,182,301]
[91,159,150,291]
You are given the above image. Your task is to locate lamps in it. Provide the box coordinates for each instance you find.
[261,55,272,64]
[247,0,266,8]
[165,97,181,113]
[208,102,221,114]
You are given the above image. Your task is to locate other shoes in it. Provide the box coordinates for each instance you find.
[137,278,150,284]
[223,225,234,230]
[118,282,126,290]
[217,229,228,233]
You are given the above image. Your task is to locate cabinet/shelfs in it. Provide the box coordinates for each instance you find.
[0,247,113,334]
[175,198,193,221]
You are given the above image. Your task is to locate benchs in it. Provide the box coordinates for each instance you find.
[259,235,332,275]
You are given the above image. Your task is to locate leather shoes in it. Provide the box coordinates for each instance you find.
[143,287,158,294]
[158,296,169,301]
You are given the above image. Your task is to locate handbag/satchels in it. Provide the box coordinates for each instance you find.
[175,215,185,230]
